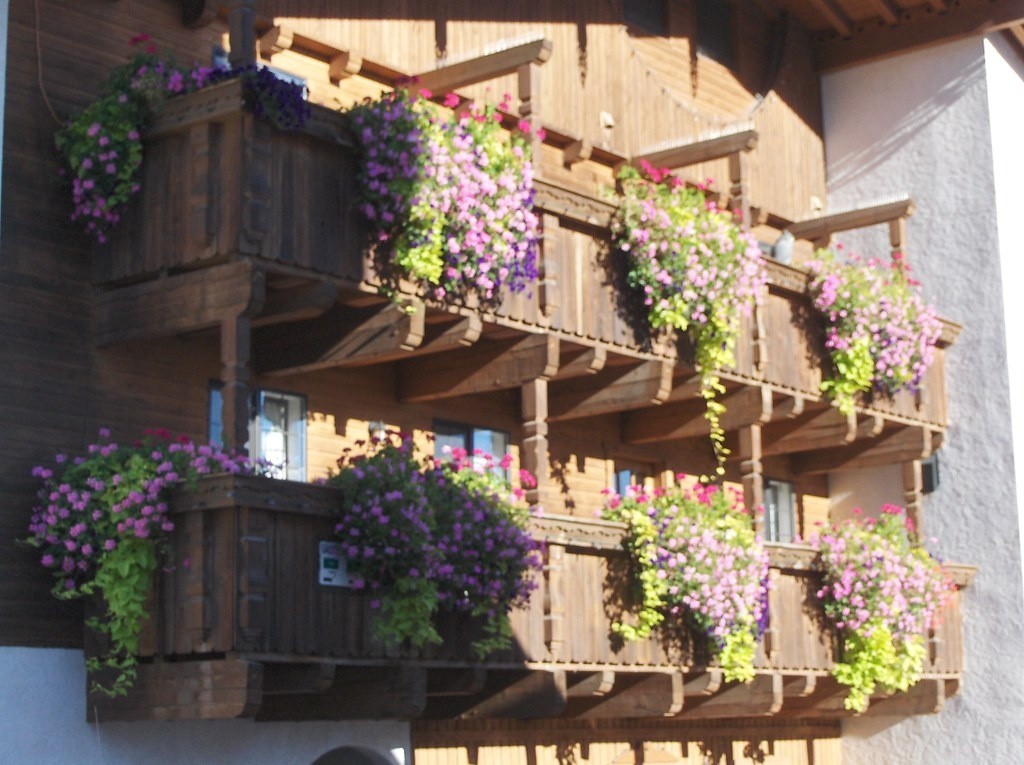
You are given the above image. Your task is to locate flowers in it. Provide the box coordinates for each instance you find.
[310,425,549,656]
[54,33,310,244]
[611,159,769,475]
[791,503,958,714]
[601,472,775,684]
[803,242,942,415]
[15,428,285,699]
[344,75,547,314]
